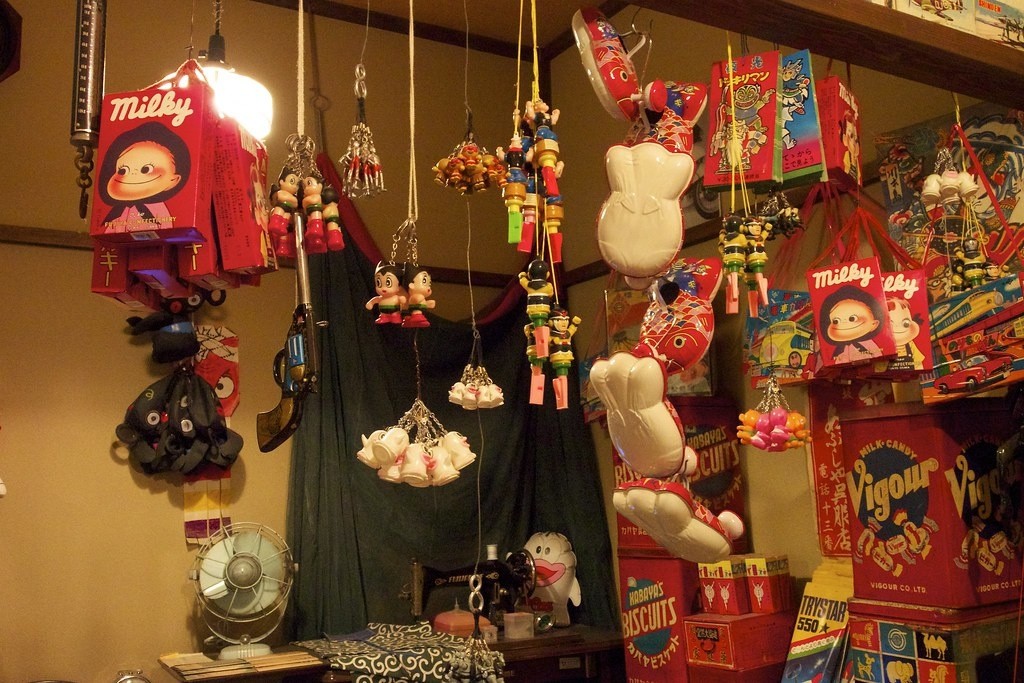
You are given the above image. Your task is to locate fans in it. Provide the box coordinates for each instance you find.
[189,520,298,660]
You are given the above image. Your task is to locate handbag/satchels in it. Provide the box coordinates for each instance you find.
[805,207,934,383]
[88,58,280,307]
[917,271,1024,406]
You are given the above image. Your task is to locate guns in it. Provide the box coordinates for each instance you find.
[254,211,322,454]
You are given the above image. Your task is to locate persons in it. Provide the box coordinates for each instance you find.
[519,260,581,367]
[367,261,435,326]
[950,236,1009,297]
[339,141,387,199]
[716,216,771,275]
[433,145,511,196]
[497,100,564,224]
[269,166,344,257]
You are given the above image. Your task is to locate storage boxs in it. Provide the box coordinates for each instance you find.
[619,558,787,683]
[806,257,933,382]
[682,608,798,672]
[504,612,534,639]
[841,396,1023,609]
[850,614,1024,683]
[613,407,748,544]
[702,47,862,195]
[89,85,279,313]
[698,551,794,615]
